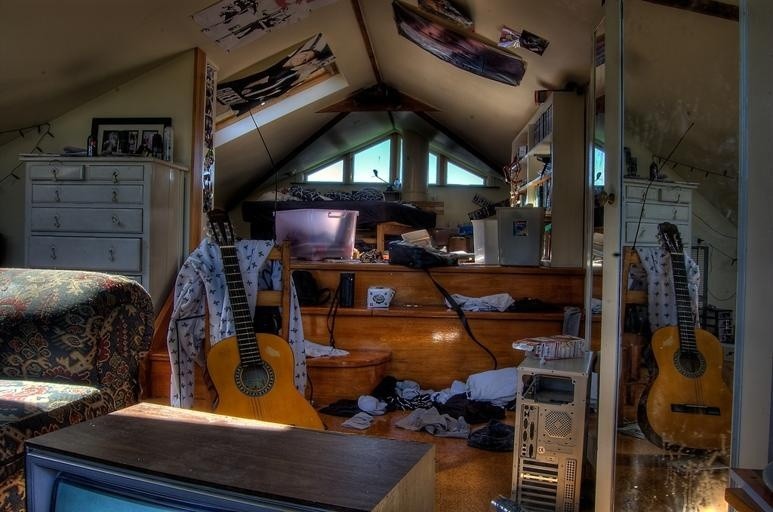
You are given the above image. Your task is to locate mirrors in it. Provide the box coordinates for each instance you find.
[584,15,608,485]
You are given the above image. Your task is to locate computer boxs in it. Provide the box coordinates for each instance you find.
[510,350,594,512]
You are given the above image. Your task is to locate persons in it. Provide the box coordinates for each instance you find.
[102,132,126,155]
[230,49,319,109]
[403,11,499,71]
[201,64,214,214]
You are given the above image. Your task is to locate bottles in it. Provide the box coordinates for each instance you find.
[649,160,659,182]
[87,127,97,157]
[152,120,174,163]
[339,271,355,307]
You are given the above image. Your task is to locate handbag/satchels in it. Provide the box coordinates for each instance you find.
[388,239,459,270]
[291,270,331,307]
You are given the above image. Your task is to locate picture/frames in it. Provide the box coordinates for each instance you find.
[91,118,171,160]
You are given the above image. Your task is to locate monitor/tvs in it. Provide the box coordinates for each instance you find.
[23,402,436,512]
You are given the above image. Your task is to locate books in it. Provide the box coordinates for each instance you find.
[507,104,553,259]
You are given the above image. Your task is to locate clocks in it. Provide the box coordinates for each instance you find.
[608,0,748,511]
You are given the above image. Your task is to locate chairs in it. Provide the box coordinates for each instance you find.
[0,265,154,512]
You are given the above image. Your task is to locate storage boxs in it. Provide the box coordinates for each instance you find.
[495,207,544,266]
[471,220,499,265]
[459,224,473,235]
[272,208,360,262]
[448,237,473,253]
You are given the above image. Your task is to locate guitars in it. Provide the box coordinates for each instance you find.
[634,222,732,454]
[203,209,326,433]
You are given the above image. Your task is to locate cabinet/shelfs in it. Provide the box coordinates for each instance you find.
[18,156,189,319]
[511,90,584,268]
[624,177,700,248]
